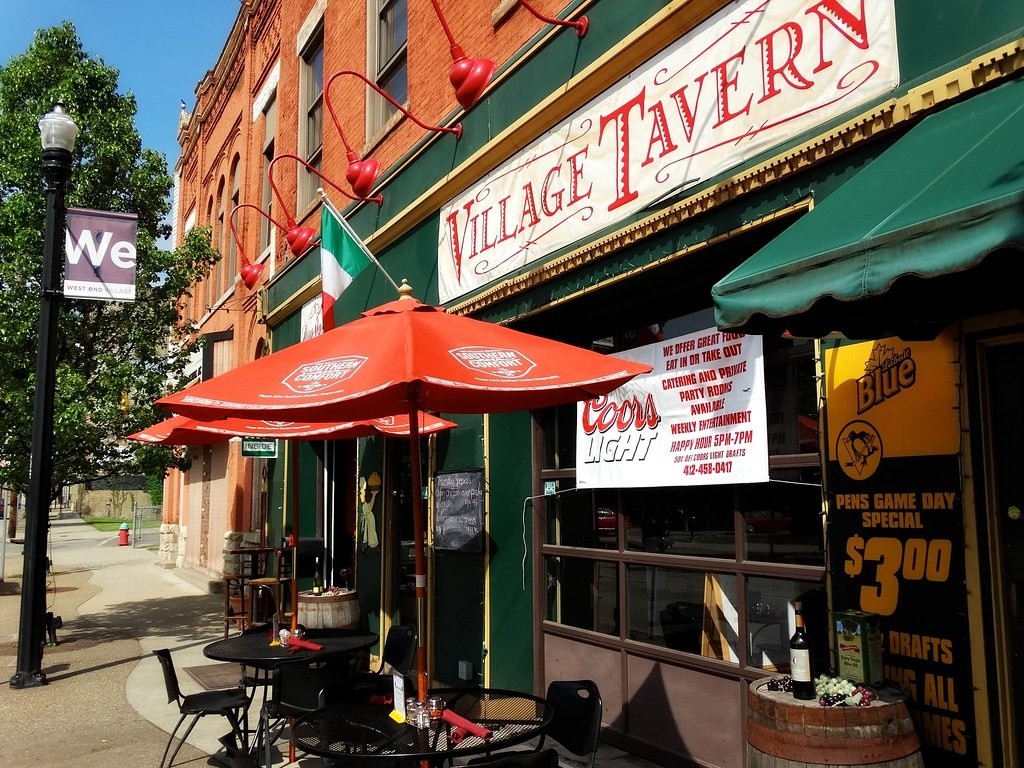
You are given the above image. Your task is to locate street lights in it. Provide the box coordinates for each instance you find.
[10,106,83,692]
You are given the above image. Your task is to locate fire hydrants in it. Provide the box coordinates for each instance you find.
[118,521,131,547]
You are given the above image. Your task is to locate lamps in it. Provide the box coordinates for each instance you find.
[268,153,385,261]
[430,1,590,114]
[323,69,464,203]
[229,203,322,292]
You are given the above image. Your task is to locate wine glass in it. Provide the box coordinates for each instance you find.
[340,568,352,592]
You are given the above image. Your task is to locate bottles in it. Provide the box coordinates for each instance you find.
[416,702,430,724]
[312,557,323,596]
[789,602,817,700]
[406,697,417,720]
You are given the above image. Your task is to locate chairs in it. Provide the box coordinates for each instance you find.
[274,619,603,767]
[152,646,251,768]
[246,547,292,628]
[223,547,277,642]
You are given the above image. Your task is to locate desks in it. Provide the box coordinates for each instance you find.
[289,686,556,767]
[204,625,380,757]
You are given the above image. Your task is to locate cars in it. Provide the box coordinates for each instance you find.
[596,507,632,536]
[0,499,4,517]
